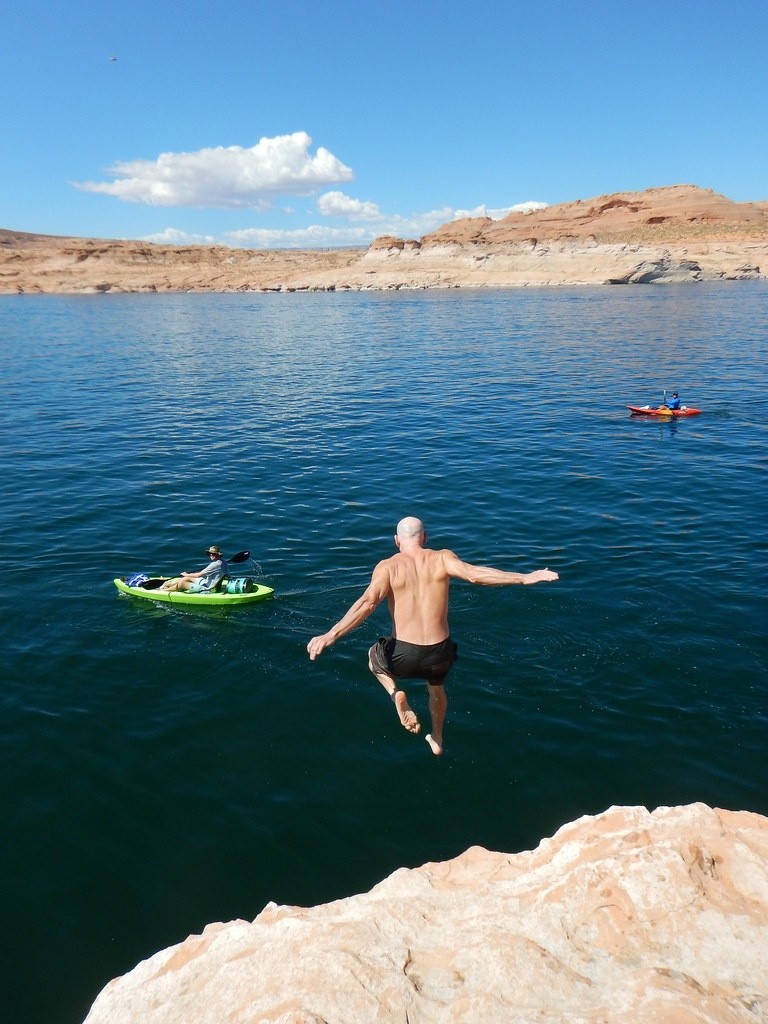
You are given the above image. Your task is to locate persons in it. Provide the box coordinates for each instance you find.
[665,391,680,410]
[159,546,230,592]
[306,512,560,755]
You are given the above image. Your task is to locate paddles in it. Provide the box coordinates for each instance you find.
[663,390,666,405]
[140,550,251,590]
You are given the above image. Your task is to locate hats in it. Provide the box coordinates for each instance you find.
[206,545,223,555]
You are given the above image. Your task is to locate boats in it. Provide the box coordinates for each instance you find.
[627,403,700,417]
[115,576,273,604]
